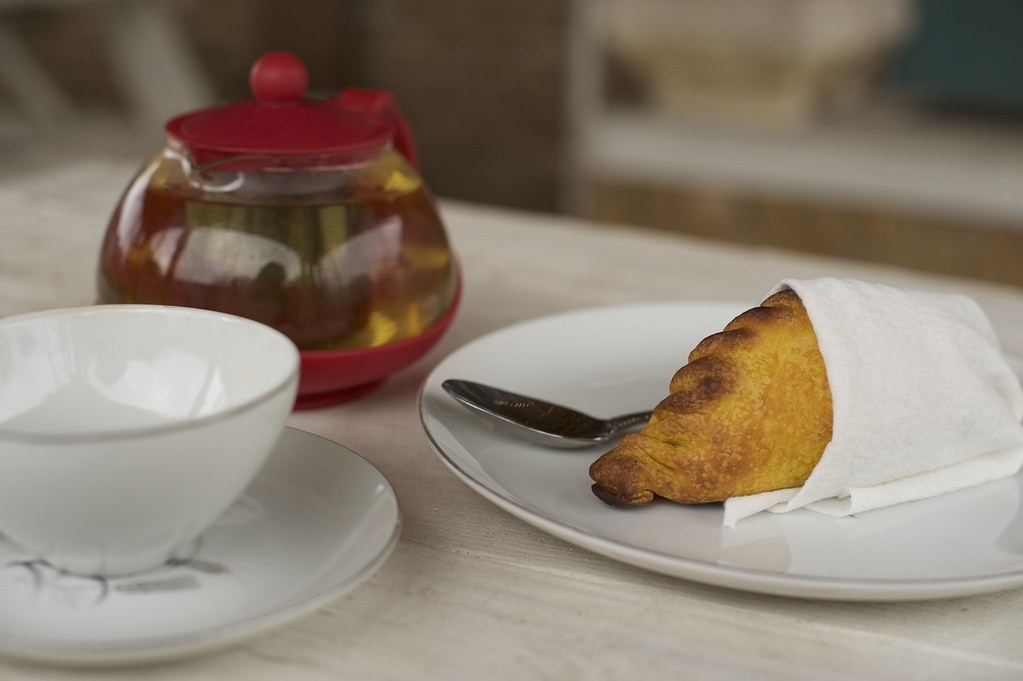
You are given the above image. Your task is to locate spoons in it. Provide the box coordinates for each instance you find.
[441,380,653,451]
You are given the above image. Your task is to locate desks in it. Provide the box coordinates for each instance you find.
[0,153,1023,681]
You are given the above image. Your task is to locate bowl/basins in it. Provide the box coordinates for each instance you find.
[1,304,301,579]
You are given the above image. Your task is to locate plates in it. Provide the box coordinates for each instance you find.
[0,425,402,671]
[419,301,1022,602]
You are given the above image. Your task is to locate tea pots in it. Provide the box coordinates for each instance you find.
[97,52,460,412]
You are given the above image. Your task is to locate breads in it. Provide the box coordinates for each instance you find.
[588,290,833,504]
